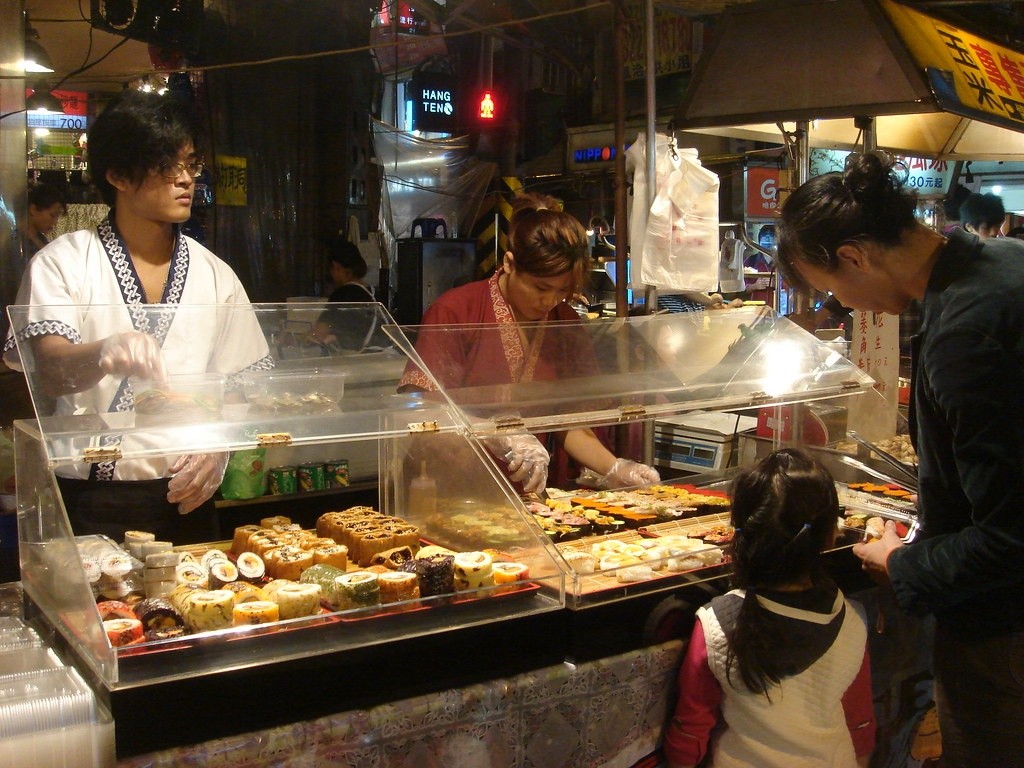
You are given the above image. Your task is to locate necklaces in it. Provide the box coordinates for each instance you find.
[131,237,175,307]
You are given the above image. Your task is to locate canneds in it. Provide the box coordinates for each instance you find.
[324,459,350,488]
[269,465,297,494]
[297,462,325,492]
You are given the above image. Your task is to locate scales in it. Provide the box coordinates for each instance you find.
[654,413,757,474]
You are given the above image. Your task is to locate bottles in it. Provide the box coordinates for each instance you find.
[447,208,458,240]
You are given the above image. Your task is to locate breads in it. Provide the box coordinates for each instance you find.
[836,434,919,463]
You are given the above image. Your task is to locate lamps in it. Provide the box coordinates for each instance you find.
[22,9,56,73]
[25,79,65,115]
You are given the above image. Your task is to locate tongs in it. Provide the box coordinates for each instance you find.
[837,429,918,495]
[504,449,552,500]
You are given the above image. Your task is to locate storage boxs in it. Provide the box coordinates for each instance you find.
[262,353,409,491]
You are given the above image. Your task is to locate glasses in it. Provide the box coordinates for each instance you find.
[147,162,205,180]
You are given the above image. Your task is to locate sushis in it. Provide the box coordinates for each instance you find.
[424,485,738,582]
[66,505,529,655]
[844,483,914,544]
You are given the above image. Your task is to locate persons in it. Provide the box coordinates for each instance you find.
[0,183,67,419]
[658,449,875,768]
[776,153,1024,768]
[277,241,389,360]
[2,94,276,549]
[956,194,1007,249]
[738,225,786,311]
[385,193,663,517]
[787,293,857,352]
[942,183,972,236]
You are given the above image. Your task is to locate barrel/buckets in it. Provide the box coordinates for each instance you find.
[288,297,328,335]
[323,459,350,490]
[268,466,298,495]
[297,461,325,493]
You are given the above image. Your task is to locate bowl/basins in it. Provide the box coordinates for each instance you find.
[840,498,920,544]
[242,366,351,415]
[127,372,228,418]
